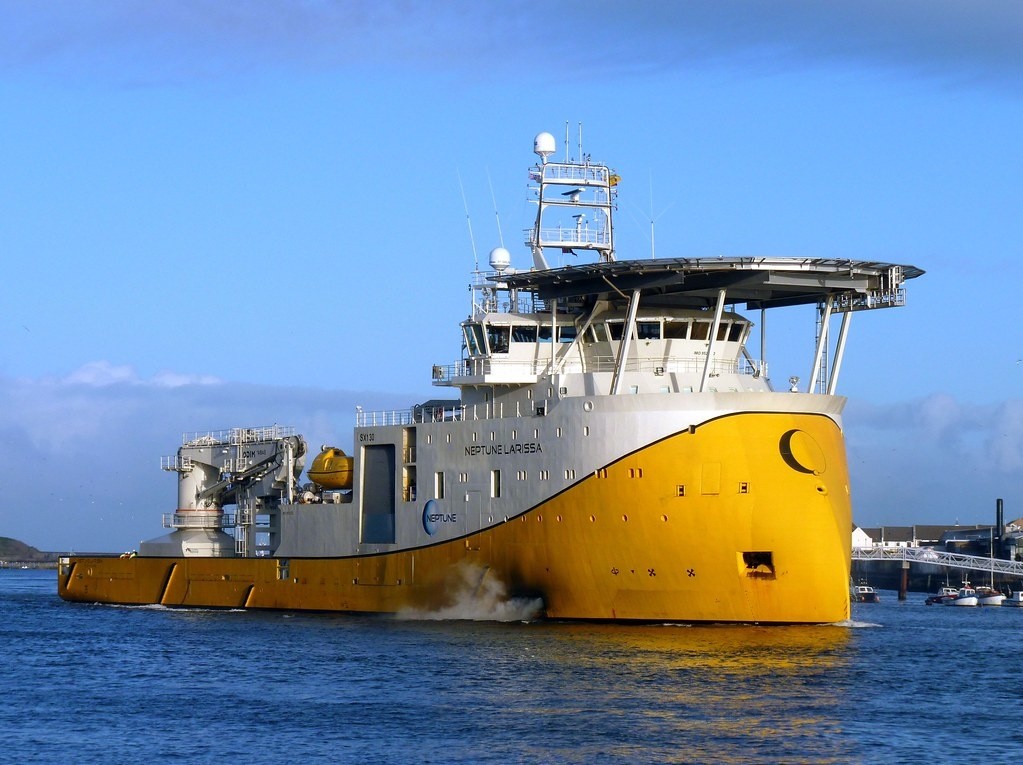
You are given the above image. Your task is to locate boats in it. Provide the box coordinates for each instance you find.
[1001,591,1023,607]
[57,119,928,629]
[850,585,880,603]
[928,584,959,603]
[943,574,979,606]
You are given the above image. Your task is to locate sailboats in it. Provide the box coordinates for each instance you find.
[975,526,1007,605]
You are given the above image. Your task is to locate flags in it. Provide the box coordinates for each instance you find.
[603,175,622,186]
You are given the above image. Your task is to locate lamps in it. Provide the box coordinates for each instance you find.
[559,387,567,398]
[656,367,664,376]
[752,370,761,379]
[789,375,801,393]
